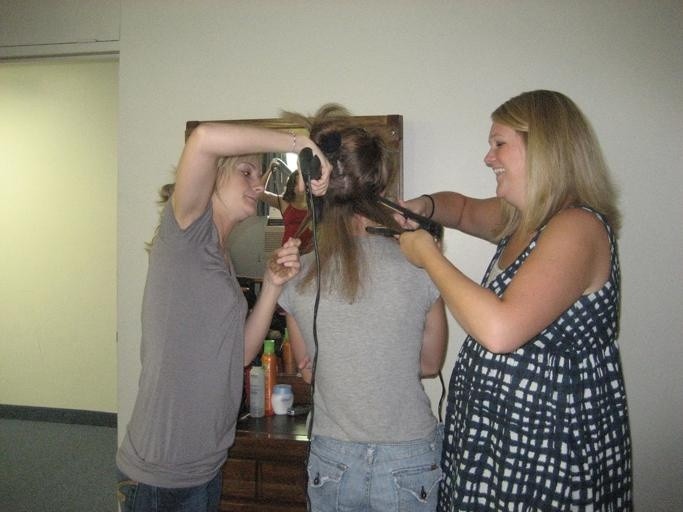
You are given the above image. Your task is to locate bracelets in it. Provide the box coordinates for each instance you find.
[288,130,297,154]
[420,194,435,220]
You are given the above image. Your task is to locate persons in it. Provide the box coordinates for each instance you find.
[114,122,334,510]
[389,89,634,512]
[269,101,446,510]
[259,158,314,256]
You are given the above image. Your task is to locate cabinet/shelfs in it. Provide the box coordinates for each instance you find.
[219,405,307,511]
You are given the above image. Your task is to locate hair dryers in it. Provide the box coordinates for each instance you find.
[270,159,291,180]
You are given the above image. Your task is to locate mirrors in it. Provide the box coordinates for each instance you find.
[229,129,317,374]
